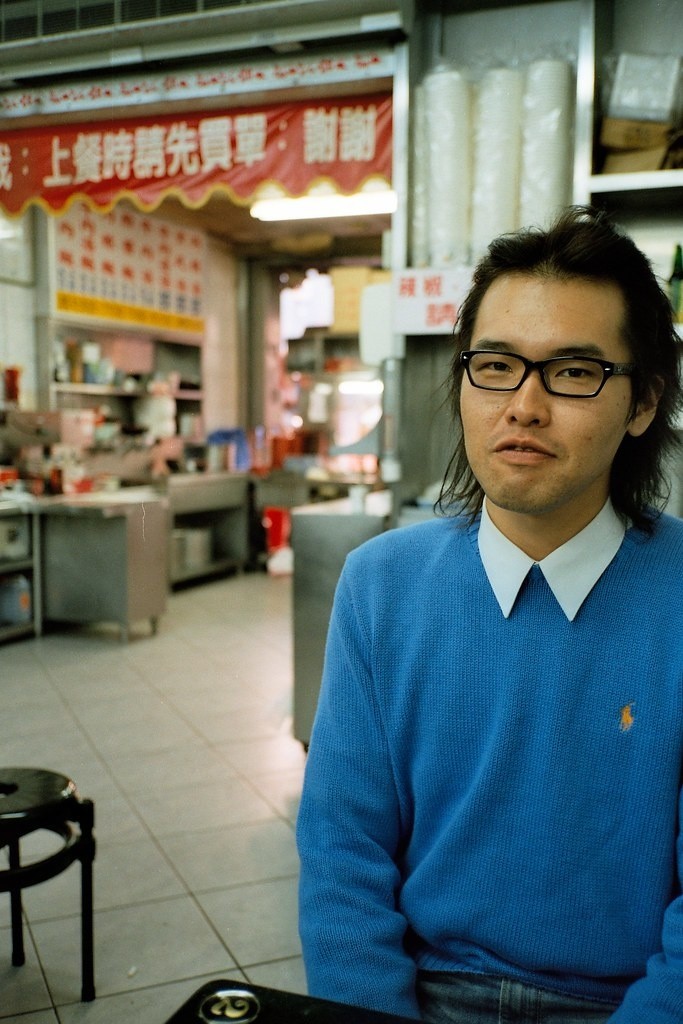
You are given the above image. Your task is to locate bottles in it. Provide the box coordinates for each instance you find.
[0,576,30,625]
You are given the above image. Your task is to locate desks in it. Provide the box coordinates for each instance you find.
[291,488,393,756]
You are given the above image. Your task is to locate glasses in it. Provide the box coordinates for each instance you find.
[460,350,633,398]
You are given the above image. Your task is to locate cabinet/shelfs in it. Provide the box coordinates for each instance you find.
[0,315,256,650]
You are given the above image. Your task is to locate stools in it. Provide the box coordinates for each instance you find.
[0,768,97,1005]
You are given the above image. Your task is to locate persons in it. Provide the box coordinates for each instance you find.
[295,209,683,1024]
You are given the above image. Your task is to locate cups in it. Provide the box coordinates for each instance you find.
[349,486,367,513]
[411,58,568,270]
